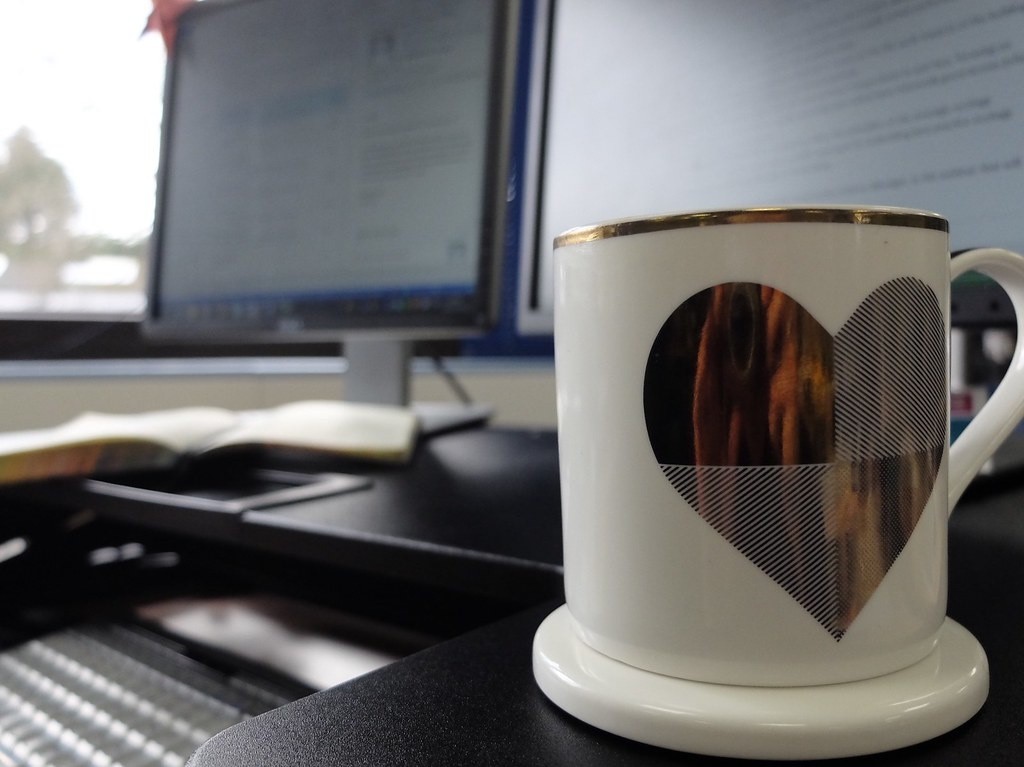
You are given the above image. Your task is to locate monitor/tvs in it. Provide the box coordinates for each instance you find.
[144,0,525,437]
[518,0,1024,337]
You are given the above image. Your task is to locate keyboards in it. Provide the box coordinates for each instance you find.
[0,609,310,767]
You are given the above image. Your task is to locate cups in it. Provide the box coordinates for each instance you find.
[552,206,1024,686]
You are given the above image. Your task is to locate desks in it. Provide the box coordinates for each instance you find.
[0,417,1024,767]
